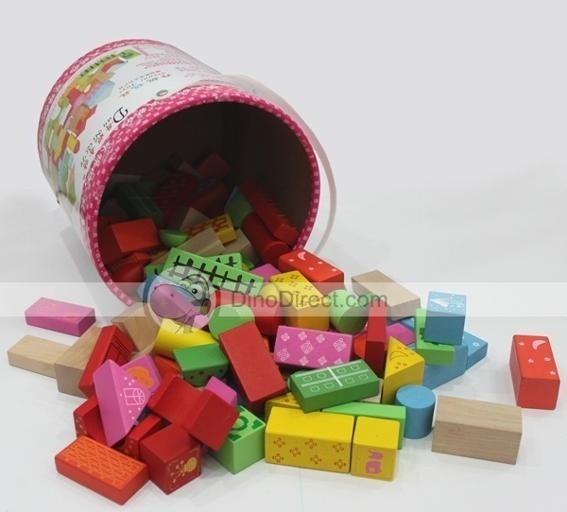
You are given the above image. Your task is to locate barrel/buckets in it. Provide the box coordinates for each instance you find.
[36,39,337,304]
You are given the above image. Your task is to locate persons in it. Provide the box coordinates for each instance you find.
[8,140,560,506]
